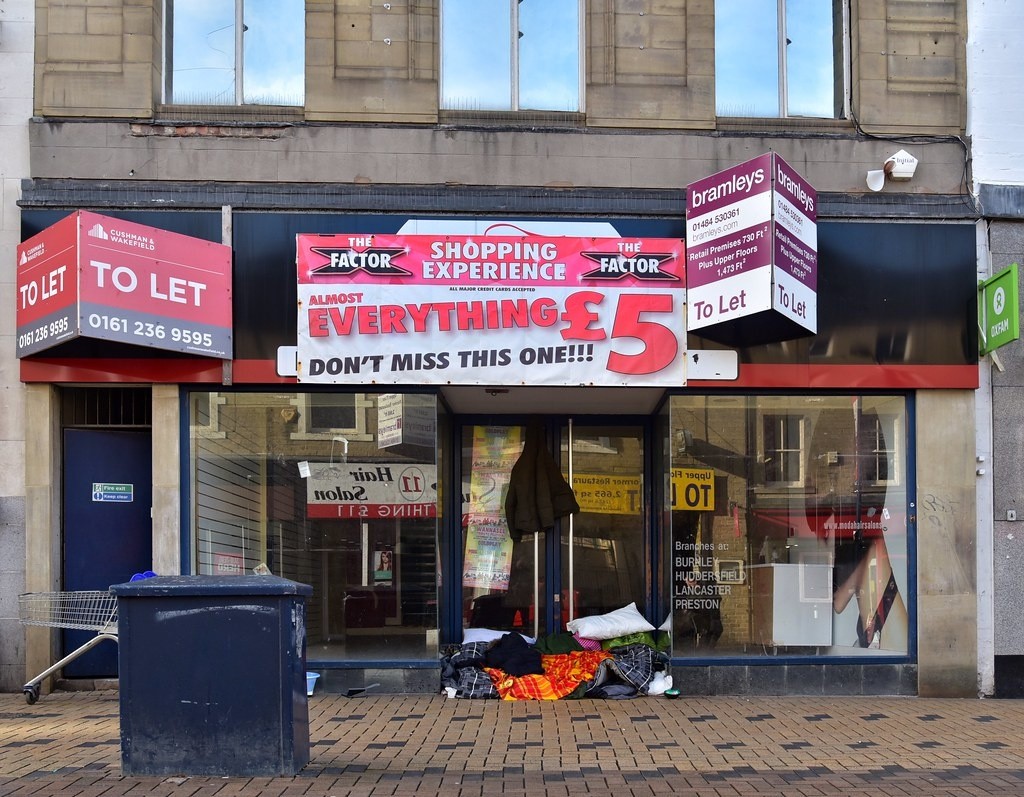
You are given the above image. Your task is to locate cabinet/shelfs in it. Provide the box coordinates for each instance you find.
[735,564,835,656]
